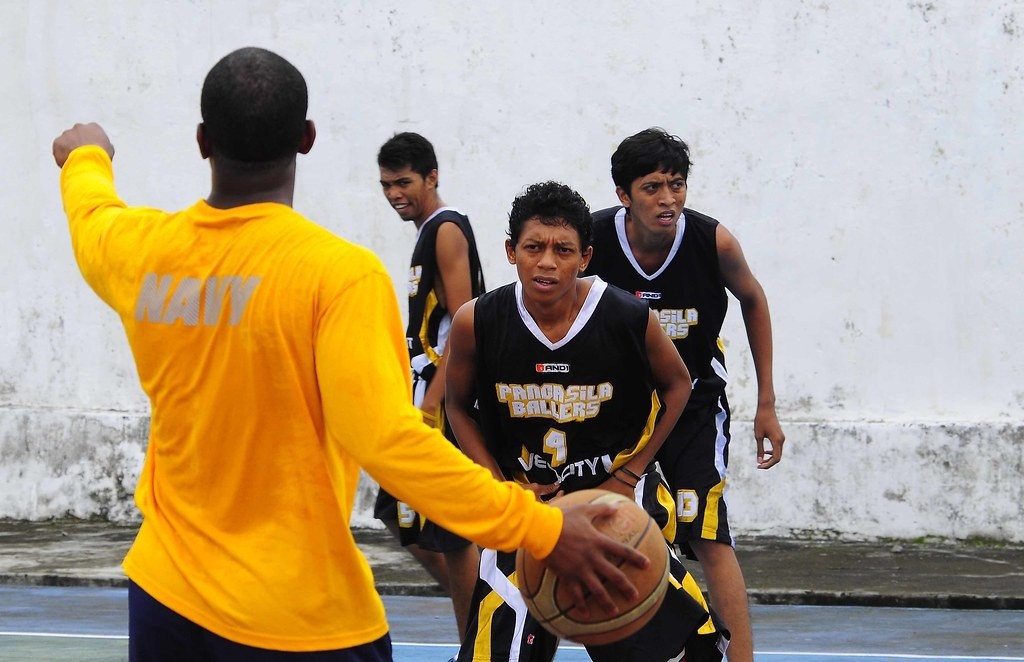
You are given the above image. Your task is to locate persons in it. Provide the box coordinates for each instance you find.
[372,127,786,662]
[52,45,651,662]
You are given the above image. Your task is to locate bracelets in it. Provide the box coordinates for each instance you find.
[612,473,637,489]
[620,465,641,481]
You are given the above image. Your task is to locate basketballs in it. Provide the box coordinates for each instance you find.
[513,488,673,649]
